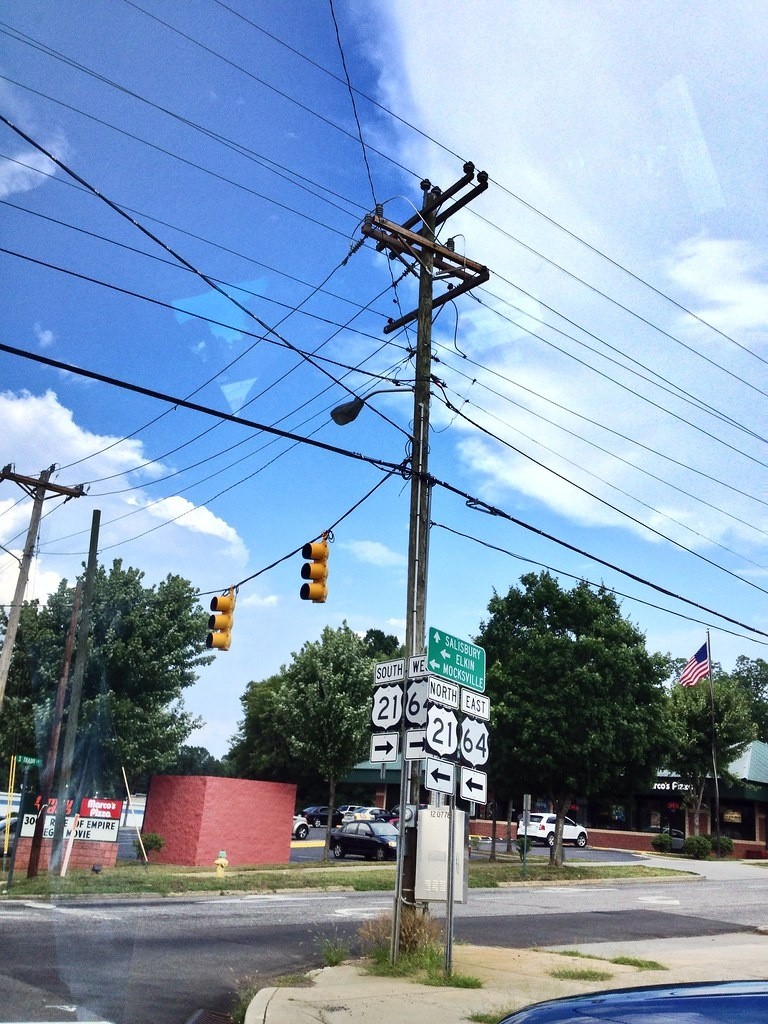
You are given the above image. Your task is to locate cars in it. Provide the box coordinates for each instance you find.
[292,816,310,840]
[329,819,399,862]
[643,826,685,853]
[337,804,400,833]
[299,806,345,828]
[0,792,22,856]
[496,979,768,1024]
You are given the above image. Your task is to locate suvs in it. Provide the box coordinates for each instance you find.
[516,813,588,848]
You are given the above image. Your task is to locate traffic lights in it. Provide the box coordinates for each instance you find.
[205,593,237,650]
[298,541,329,605]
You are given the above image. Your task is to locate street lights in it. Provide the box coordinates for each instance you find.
[330,388,424,960]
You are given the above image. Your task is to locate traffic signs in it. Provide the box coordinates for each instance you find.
[426,625,487,693]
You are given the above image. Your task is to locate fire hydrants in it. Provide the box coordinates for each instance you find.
[215,849,229,879]
